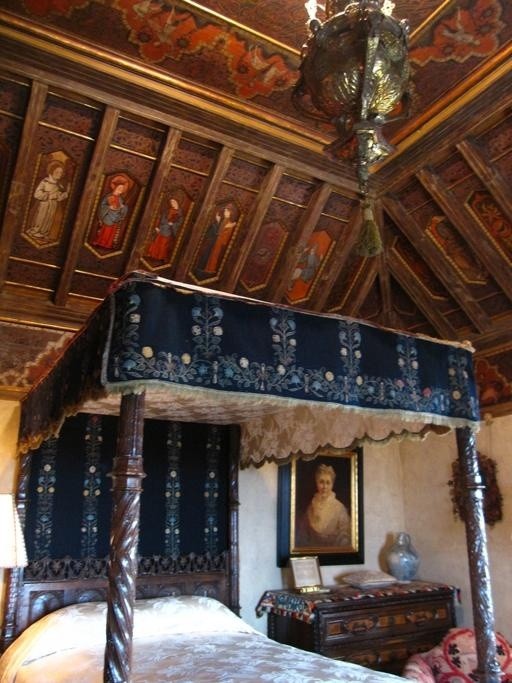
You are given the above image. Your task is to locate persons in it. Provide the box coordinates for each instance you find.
[296,464,351,546]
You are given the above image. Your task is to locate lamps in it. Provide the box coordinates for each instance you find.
[1,493,29,568]
[292,1,416,257]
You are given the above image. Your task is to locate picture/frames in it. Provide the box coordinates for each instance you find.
[290,556,323,589]
[276,446,364,568]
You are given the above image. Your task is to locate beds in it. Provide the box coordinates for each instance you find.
[1,572,416,683]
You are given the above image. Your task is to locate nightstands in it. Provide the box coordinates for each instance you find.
[255,579,462,676]
